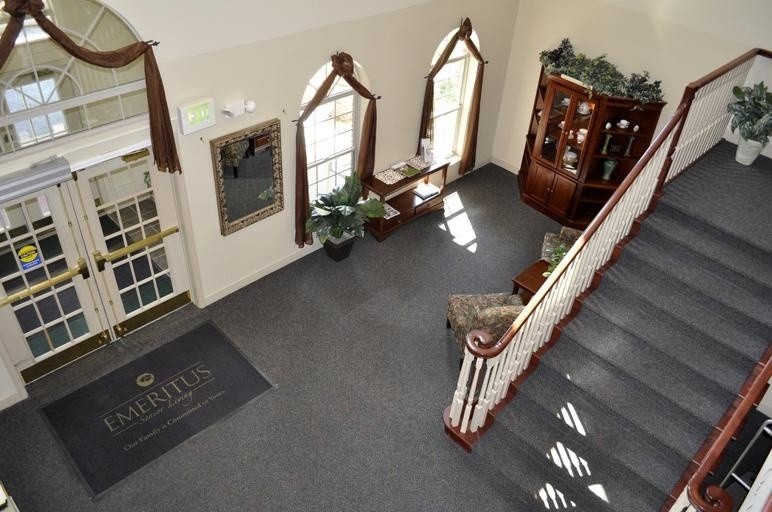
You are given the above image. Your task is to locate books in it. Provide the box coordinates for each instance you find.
[375,155,438,220]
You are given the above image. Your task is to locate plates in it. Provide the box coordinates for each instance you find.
[616,123,629,129]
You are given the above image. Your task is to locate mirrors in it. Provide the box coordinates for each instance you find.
[210,117,285,237]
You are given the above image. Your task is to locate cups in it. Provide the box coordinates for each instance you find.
[563,98,570,104]
[562,151,578,166]
[620,119,630,126]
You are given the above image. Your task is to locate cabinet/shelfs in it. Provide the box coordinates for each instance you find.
[517,59,668,232]
[364,154,451,242]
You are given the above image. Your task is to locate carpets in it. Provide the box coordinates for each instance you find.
[36,318,281,498]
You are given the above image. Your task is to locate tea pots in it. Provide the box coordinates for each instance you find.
[577,98,590,115]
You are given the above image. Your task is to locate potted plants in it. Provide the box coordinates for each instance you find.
[728,80,772,165]
[307,172,387,263]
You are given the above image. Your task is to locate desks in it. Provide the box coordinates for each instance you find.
[250,134,272,157]
[511,257,553,305]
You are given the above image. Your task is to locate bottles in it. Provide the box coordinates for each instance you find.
[601,159,618,182]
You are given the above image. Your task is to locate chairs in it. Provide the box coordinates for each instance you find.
[220,139,250,180]
[540,223,585,264]
[445,289,527,369]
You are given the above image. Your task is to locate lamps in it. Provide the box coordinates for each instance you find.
[219,97,257,118]
